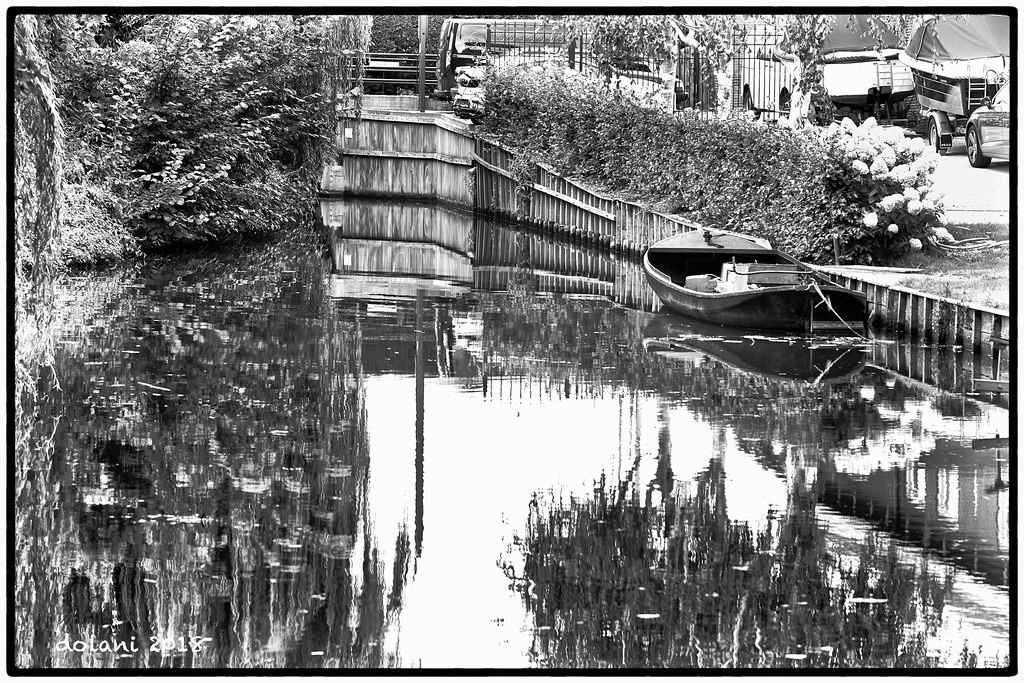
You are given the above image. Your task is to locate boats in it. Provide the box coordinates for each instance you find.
[642,228,865,337]
[642,304,868,392]
[897,14,1009,119]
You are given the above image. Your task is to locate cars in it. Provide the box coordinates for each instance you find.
[556,49,689,104]
[453,47,558,125]
[965,75,1010,167]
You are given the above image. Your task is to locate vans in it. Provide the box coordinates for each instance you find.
[432,17,592,105]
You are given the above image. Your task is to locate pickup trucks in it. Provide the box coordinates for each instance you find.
[738,14,915,125]
